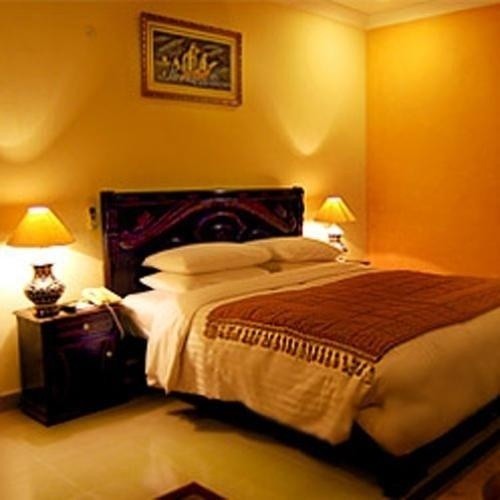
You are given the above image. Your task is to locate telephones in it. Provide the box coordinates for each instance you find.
[82,287,122,307]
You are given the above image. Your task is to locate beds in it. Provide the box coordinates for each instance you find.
[98,186,500,500]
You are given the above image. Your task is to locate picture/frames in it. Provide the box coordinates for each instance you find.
[139,10,243,107]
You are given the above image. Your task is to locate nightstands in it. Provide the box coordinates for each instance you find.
[13,299,129,427]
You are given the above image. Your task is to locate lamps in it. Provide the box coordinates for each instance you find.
[314,196,358,260]
[6,206,76,318]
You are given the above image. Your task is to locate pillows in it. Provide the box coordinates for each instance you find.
[138,236,341,294]
